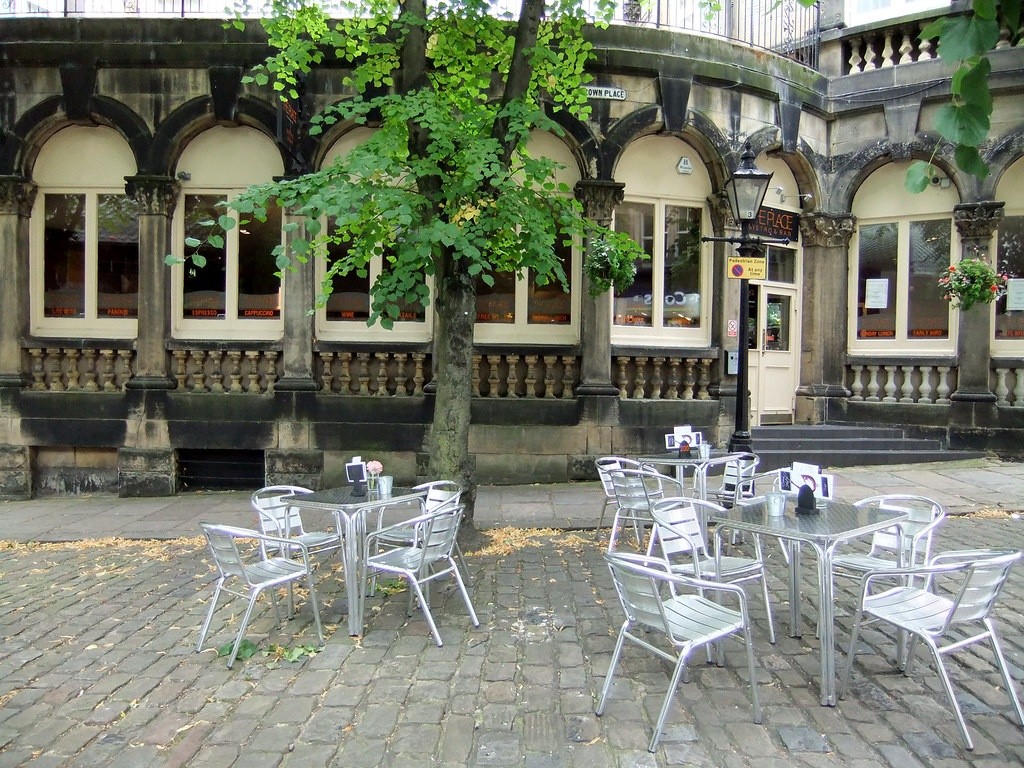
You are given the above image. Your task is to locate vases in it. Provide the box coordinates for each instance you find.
[367,473,379,491]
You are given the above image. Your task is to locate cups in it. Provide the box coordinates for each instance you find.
[378,476,393,495]
[765,492,787,517]
[696,444,712,459]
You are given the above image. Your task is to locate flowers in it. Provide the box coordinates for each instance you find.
[938,259,1007,311]
[367,460,383,487]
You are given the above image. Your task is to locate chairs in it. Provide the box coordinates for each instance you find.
[359,504,480,647]
[196,523,323,669]
[370,481,473,596]
[251,485,350,646]
[594,454,1024,750]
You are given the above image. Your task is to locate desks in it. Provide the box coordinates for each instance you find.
[708,497,909,707]
[280,486,427,634]
[638,451,743,555]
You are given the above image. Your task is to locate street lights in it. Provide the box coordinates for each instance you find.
[702,142,788,518]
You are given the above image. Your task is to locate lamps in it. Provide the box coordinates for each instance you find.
[767,187,783,195]
[781,194,812,202]
